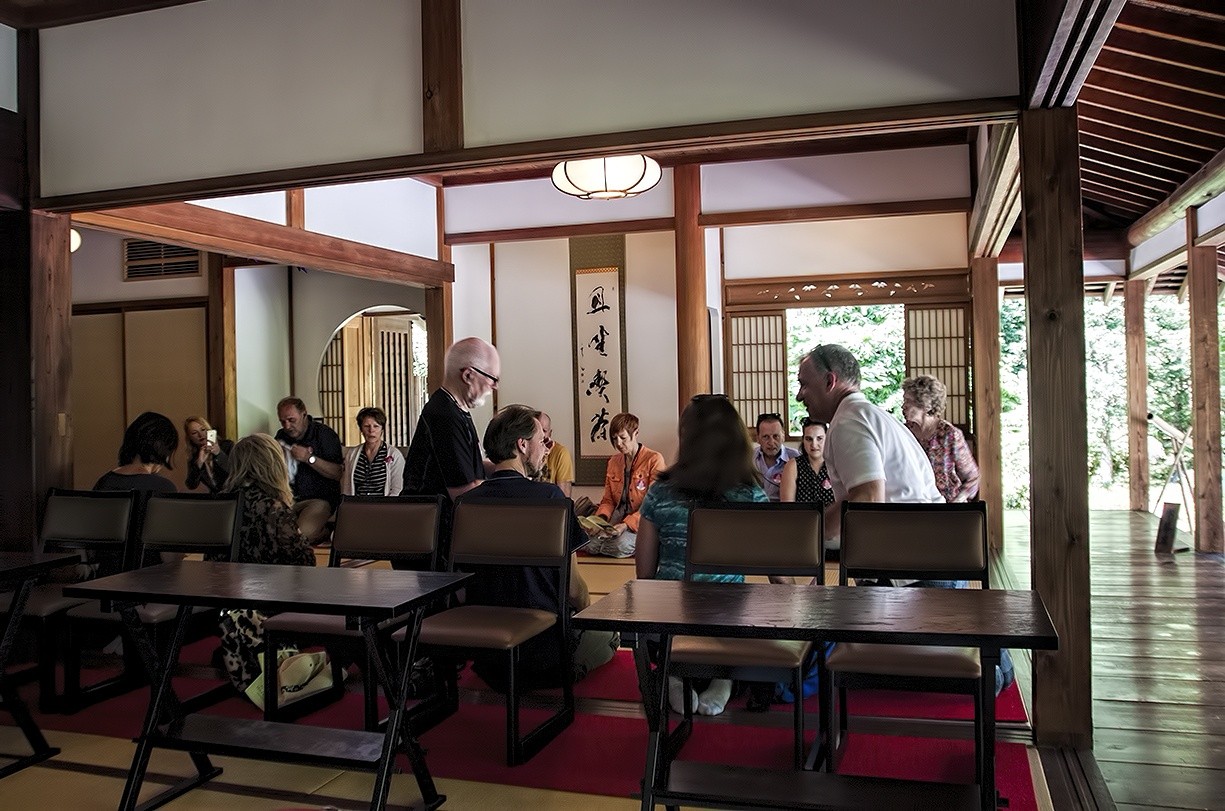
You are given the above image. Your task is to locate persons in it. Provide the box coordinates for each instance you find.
[202,433,316,706]
[780,418,835,509]
[341,406,406,497]
[795,345,1013,696]
[527,411,572,499]
[184,415,234,494]
[585,413,665,559]
[274,397,343,544]
[399,338,496,573]
[637,393,795,716]
[93,412,189,576]
[901,374,981,505]
[751,413,802,505]
[450,404,621,688]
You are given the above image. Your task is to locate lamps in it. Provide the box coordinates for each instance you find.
[71,228,82,254]
[551,155,663,201]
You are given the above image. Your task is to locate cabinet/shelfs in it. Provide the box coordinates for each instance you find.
[70,301,212,494]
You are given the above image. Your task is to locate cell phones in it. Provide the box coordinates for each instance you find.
[207,430,217,446]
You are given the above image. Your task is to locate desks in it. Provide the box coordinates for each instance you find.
[62,559,475,811]
[569,579,1059,811]
[0,549,82,781]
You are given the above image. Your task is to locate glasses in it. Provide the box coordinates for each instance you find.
[757,412,781,421]
[459,366,499,386]
[810,343,838,383]
[690,394,730,402]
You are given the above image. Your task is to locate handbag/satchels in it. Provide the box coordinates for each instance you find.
[242,645,350,715]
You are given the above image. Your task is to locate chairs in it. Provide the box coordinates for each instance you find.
[0,489,990,801]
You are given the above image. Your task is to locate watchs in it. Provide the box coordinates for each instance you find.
[307,455,317,465]
